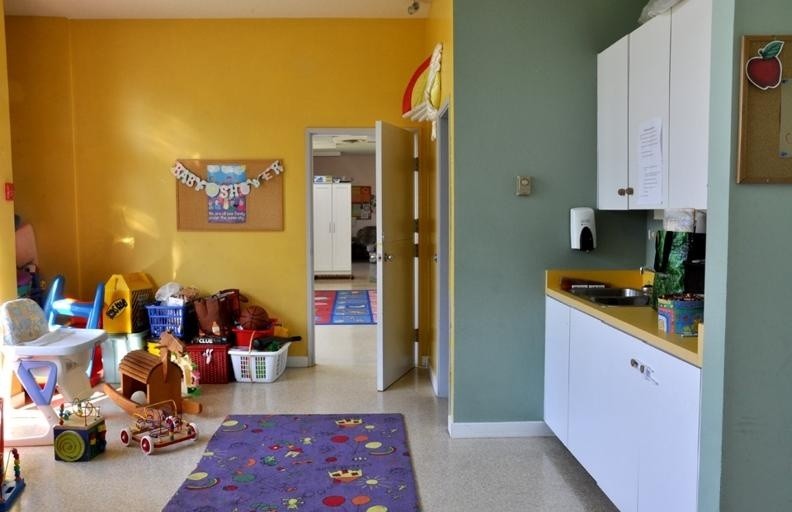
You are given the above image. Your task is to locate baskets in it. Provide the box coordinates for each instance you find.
[228,342,291,383]
[186,343,228,385]
[147,304,194,339]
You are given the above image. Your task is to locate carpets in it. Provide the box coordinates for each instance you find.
[161,413,421,512]
[314,290,377,325]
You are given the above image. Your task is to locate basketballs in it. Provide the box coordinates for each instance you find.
[239,305,270,330]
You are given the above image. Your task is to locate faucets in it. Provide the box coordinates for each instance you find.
[638,264,658,276]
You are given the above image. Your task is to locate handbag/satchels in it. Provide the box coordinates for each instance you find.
[193,289,247,336]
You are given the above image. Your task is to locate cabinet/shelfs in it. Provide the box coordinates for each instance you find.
[596,0,711,211]
[544,295,702,512]
[312,182,352,278]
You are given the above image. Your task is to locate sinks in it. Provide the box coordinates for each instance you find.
[566,282,652,297]
[589,296,658,310]
